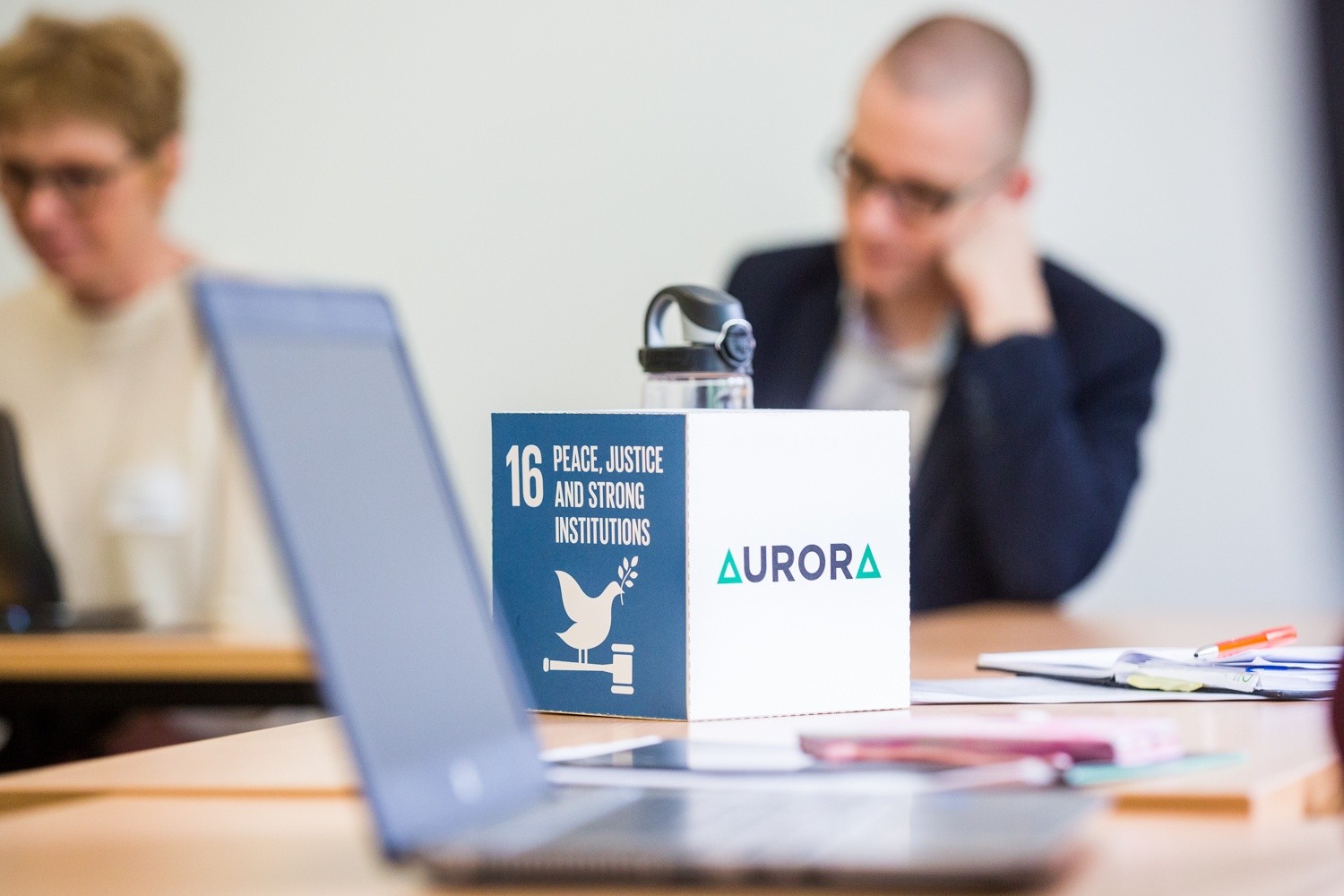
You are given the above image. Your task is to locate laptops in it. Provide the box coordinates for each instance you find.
[0,405,145,634]
[187,280,1105,888]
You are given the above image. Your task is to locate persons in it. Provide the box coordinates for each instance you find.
[0,18,315,652]
[727,19,1163,617]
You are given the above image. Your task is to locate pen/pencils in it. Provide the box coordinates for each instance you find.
[1194,626,1297,658]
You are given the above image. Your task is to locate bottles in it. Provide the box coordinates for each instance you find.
[633,283,756,409]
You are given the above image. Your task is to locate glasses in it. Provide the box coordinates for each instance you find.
[0,144,144,203]
[831,135,1006,229]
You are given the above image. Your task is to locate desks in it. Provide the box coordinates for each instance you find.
[0,590,1344,895]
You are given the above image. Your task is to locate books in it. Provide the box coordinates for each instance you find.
[976,646,1344,700]
